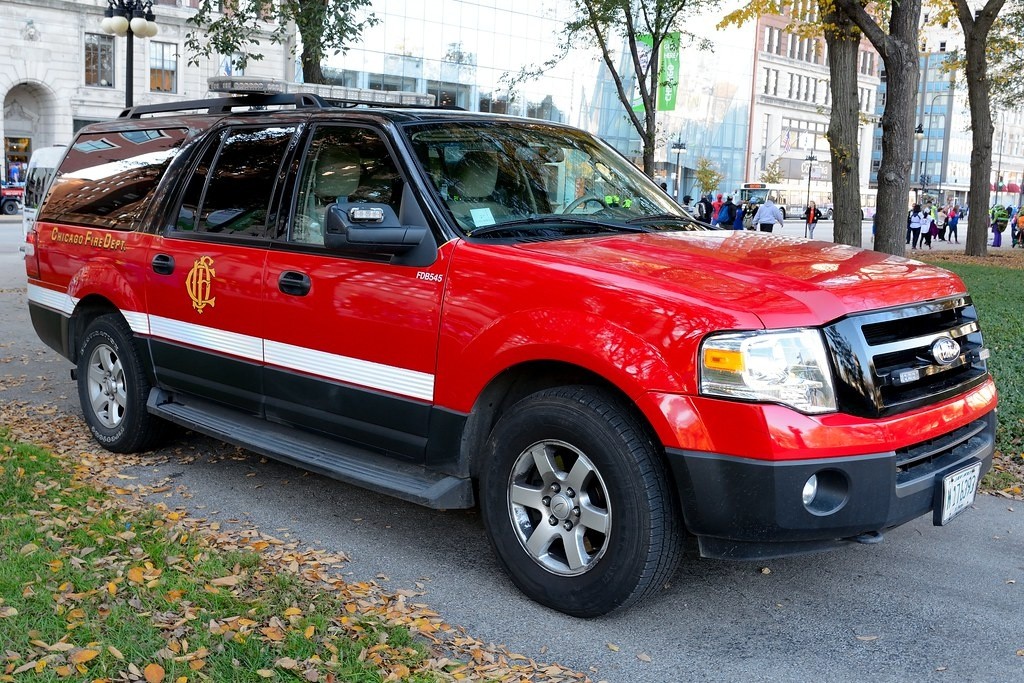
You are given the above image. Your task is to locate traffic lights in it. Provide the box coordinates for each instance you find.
[926,175,930,185]
[920,174,925,185]
[998,177,1003,187]
[923,188,928,193]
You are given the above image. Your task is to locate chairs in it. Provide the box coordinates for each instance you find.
[303,157,360,244]
[446,151,516,232]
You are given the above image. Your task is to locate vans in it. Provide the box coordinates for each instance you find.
[5,151,30,180]
[18,144,69,259]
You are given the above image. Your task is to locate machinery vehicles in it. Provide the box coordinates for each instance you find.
[0,165,24,215]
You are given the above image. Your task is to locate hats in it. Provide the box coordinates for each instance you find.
[683,196,694,201]
[924,208,930,214]
[749,197,757,204]
[727,194,733,199]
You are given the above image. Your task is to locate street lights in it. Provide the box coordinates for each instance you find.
[670,142,688,202]
[101,0,160,108]
[805,155,818,237]
[921,93,967,202]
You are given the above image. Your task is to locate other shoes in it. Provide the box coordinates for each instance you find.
[912,247,918,250]
[933,238,936,241]
[991,245,1000,247]
[906,241,910,244]
[929,247,933,251]
[938,239,942,242]
[955,241,961,244]
[947,240,954,244]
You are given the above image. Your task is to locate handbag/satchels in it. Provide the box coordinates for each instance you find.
[937,224,943,229]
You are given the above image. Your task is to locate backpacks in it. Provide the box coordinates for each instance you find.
[743,208,754,229]
[717,206,729,223]
[692,202,707,220]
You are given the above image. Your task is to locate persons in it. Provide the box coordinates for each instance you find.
[681,190,784,234]
[906,201,1024,251]
[11,162,21,183]
[804,201,822,240]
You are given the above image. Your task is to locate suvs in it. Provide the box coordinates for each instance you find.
[18,75,1001,622]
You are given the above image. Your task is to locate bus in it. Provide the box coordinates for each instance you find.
[734,181,916,221]
